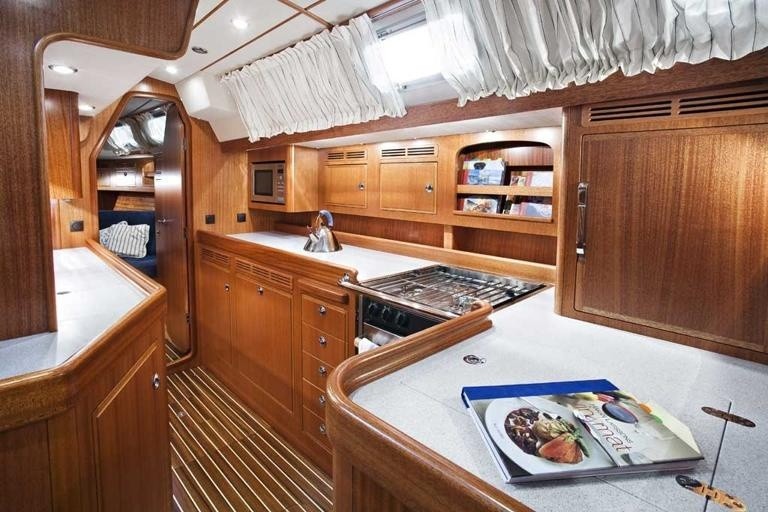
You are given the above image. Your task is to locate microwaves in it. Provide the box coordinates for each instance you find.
[249,162,285,207]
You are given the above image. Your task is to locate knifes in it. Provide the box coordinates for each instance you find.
[565,402,624,468]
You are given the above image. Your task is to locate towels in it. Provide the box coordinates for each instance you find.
[358,338,380,355]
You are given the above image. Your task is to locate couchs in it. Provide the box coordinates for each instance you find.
[97,211,157,276]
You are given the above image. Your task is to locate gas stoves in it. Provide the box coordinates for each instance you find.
[351,262,550,323]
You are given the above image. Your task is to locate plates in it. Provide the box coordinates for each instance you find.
[485,397,629,477]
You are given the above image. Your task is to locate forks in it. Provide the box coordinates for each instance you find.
[520,396,576,435]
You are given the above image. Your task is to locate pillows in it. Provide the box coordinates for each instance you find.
[98,221,149,259]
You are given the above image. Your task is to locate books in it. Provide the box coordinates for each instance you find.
[460,159,507,185]
[461,379,705,483]
[509,170,553,186]
[457,198,498,214]
[502,194,552,217]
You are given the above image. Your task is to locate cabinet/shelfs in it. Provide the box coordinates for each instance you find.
[0,319,172,512]
[320,125,560,238]
[557,79,768,367]
[197,248,354,481]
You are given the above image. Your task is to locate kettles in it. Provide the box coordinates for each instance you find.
[304,209,340,254]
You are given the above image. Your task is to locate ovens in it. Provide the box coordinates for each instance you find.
[354,294,445,358]
